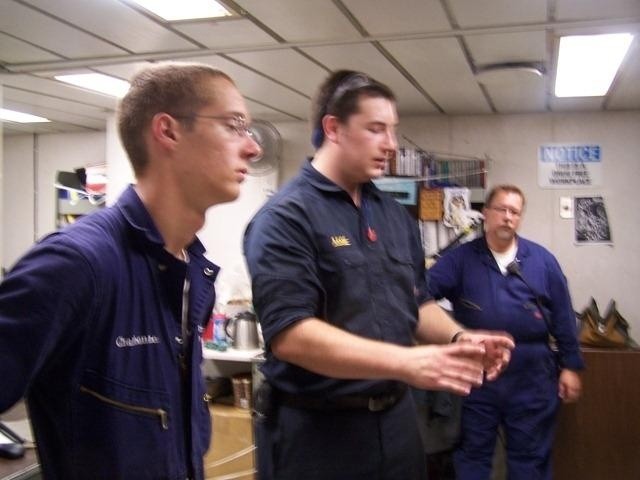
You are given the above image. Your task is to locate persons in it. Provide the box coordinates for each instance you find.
[0,61,264,480]
[425,185,585,480]
[242,71,515,480]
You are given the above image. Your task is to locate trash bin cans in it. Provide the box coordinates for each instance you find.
[231,375,251,410]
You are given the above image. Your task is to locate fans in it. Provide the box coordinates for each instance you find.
[242,118,284,177]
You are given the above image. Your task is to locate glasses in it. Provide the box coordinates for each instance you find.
[326,72,390,110]
[489,207,520,217]
[173,112,256,136]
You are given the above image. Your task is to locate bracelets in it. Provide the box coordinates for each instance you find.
[452,330,465,344]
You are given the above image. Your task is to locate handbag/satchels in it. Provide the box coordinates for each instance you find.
[576,296,630,347]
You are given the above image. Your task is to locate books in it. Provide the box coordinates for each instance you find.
[382,146,488,189]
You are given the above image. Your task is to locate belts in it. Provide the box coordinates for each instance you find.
[259,382,408,421]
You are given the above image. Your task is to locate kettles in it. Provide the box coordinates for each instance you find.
[224,311,259,351]
